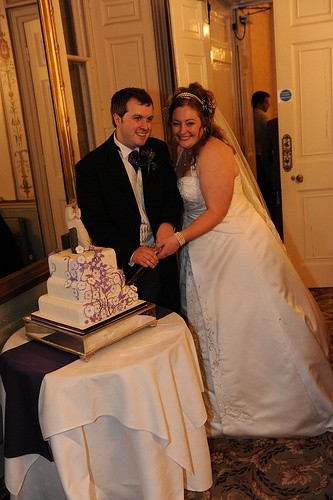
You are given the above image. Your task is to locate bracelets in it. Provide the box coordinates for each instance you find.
[173,232,186,248]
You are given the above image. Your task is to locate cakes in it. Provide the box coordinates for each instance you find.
[38,245,138,326]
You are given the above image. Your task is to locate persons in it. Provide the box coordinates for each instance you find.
[65,202,91,251]
[66,198,78,254]
[0,211,23,279]
[252,90,274,226]
[265,118,282,224]
[152,80,332,440]
[74,87,185,316]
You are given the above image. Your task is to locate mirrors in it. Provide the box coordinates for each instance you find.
[0,0,78,304]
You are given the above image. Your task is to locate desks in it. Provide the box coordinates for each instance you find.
[0,304,213,500]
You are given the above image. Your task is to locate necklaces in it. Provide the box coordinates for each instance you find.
[183,150,193,167]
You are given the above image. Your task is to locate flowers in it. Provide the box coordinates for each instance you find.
[141,147,159,175]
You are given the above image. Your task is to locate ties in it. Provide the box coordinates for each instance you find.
[128,150,140,174]
